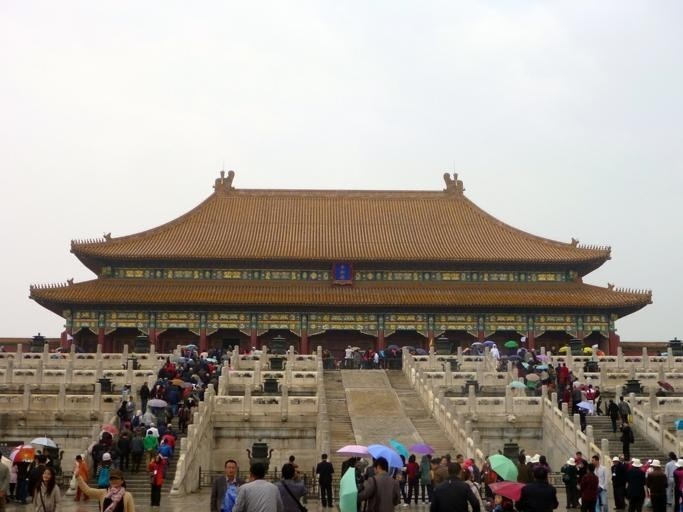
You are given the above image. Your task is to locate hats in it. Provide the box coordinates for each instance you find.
[567,458,576,466]
[632,459,642,467]
[612,457,619,461]
[526,454,540,464]
[102,453,111,461]
[110,471,123,478]
[676,459,683,467]
[650,460,661,467]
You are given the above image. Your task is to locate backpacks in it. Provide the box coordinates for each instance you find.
[98,467,110,487]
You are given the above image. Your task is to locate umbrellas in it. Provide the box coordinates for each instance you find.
[408,443,434,456]
[335,444,373,459]
[673,418,682,430]
[368,443,403,471]
[389,439,407,458]
[656,380,674,393]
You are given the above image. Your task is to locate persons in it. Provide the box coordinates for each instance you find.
[652,351,662,362]
[249,346,258,355]
[231,461,287,511]
[271,463,308,511]
[0,431,67,511]
[73,342,230,512]
[313,335,505,370]
[655,388,665,405]
[619,420,633,460]
[280,454,300,483]
[507,336,635,433]
[242,348,249,355]
[208,459,240,512]
[315,454,335,508]
[341,449,682,512]
